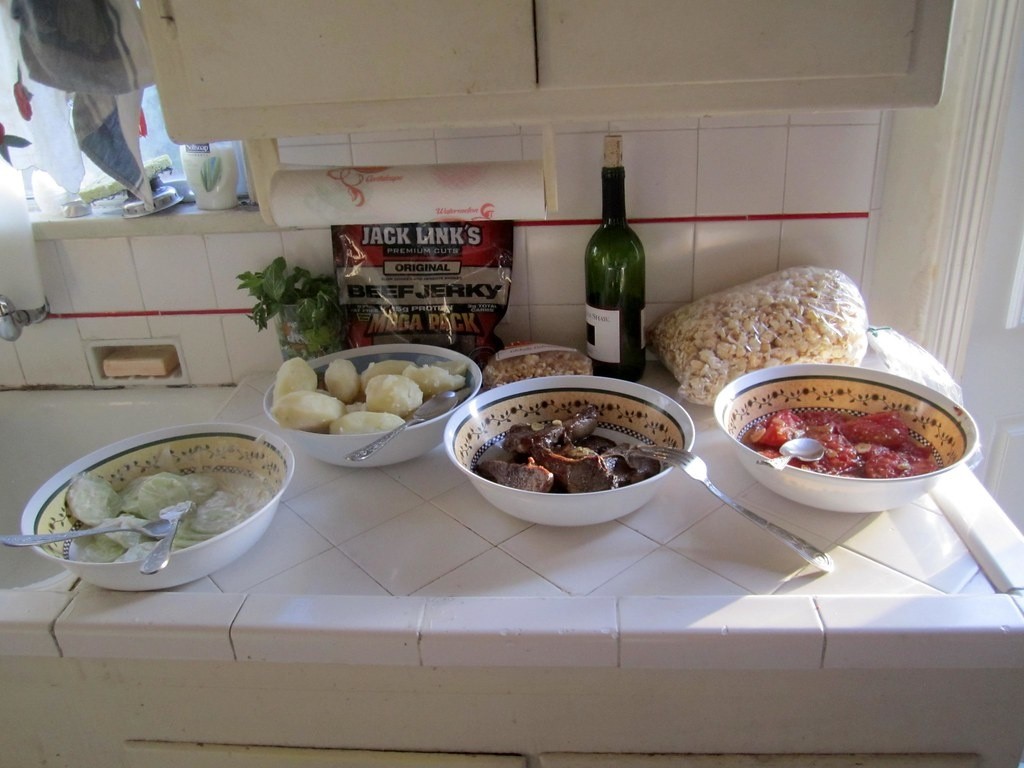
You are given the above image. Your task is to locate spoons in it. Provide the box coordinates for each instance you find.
[754,437,825,472]
[0,520,172,549]
[342,389,459,461]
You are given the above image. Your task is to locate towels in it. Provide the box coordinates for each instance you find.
[7,0,157,215]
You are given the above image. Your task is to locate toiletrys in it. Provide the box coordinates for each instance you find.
[179,141,241,211]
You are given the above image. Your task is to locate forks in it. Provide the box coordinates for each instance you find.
[139,501,198,574]
[628,444,834,573]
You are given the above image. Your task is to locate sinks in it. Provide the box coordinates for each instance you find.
[0,380,235,591]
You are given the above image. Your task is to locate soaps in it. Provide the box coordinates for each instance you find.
[101,346,179,379]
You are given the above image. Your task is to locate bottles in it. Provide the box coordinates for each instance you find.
[584,135,646,382]
[178,140,241,210]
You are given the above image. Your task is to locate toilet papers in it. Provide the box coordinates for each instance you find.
[264,159,552,234]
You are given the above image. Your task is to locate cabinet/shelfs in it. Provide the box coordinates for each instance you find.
[135,0,962,149]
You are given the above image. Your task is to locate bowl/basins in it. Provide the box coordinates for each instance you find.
[20,422,302,593]
[262,343,483,468]
[711,362,979,514]
[442,373,696,526]
